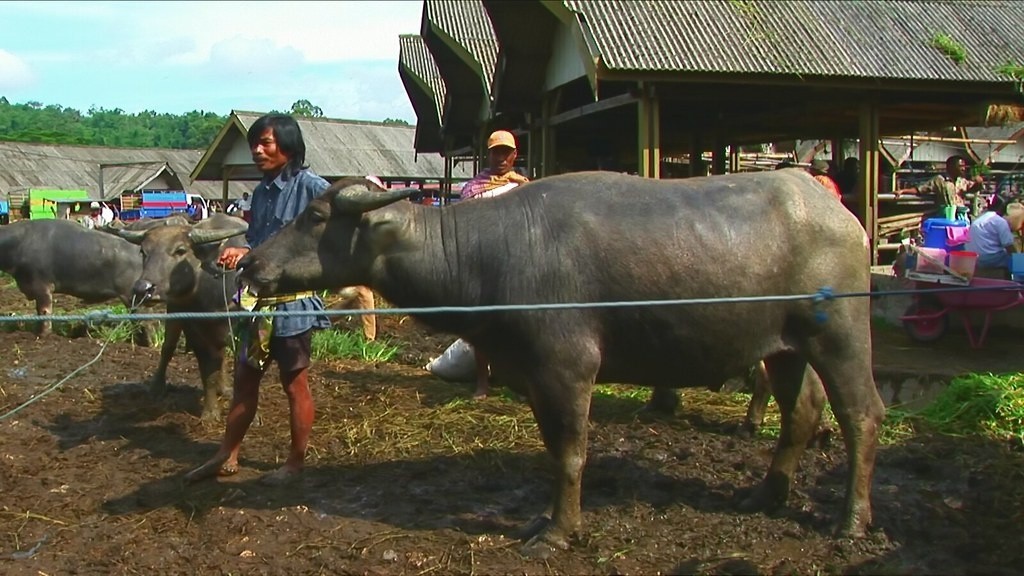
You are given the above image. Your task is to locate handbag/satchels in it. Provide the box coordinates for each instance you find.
[425,337,484,380]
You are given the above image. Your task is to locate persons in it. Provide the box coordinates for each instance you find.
[82,201,106,231]
[185,113,331,490]
[238,192,252,211]
[462,131,529,404]
[324,174,386,345]
[810,155,1024,276]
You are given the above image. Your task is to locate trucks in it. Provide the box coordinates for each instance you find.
[6,187,100,225]
[118,191,209,224]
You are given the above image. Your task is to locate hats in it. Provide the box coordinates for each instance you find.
[487,130,516,149]
[90,202,102,209]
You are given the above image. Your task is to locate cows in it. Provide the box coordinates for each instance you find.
[237,170,886,555]
[119,213,253,425]
[0,218,192,348]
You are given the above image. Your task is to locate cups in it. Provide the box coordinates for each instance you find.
[946,207,955,222]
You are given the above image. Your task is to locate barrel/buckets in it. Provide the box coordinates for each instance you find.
[916,246,946,274]
[921,217,967,274]
[949,251,979,281]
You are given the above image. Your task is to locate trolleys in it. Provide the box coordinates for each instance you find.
[897,274,1024,351]
